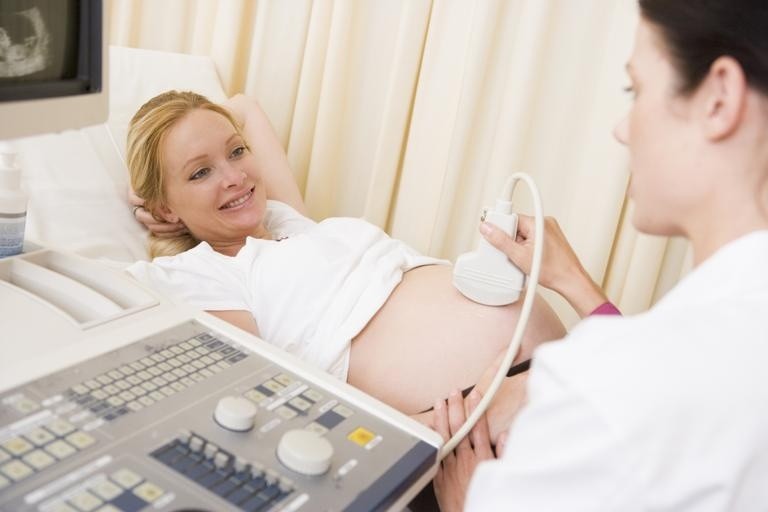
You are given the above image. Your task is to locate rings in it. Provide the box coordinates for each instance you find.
[131,205,144,214]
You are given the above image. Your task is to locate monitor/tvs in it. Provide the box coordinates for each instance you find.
[0,0,111,141]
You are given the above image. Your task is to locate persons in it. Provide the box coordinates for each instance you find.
[118,89,569,512]
[432,0,768,512]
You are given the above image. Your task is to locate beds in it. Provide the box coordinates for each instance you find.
[1,47,232,328]
